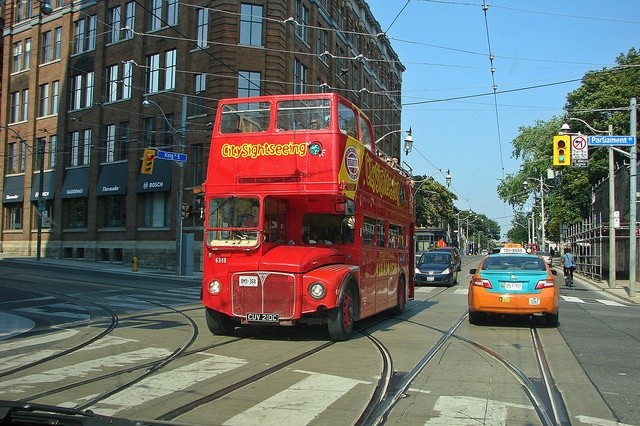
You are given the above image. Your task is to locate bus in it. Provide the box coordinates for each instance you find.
[202,91,417,342]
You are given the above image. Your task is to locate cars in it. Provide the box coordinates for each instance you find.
[429,246,461,271]
[414,252,459,287]
[536,250,552,267]
[469,244,560,325]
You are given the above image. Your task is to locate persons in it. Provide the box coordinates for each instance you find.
[561,249,574,285]
[311,120,320,129]
[385,157,399,168]
[242,201,268,234]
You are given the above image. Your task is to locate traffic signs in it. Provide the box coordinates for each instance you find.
[571,132,589,168]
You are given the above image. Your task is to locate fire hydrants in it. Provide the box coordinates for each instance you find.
[131,256,139,272]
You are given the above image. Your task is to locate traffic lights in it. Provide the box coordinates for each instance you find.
[552,137,571,166]
[140,149,156,175]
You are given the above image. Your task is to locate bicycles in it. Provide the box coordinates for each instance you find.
[564,267,573,285]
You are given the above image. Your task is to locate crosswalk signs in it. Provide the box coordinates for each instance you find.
[414,285,629,308]
